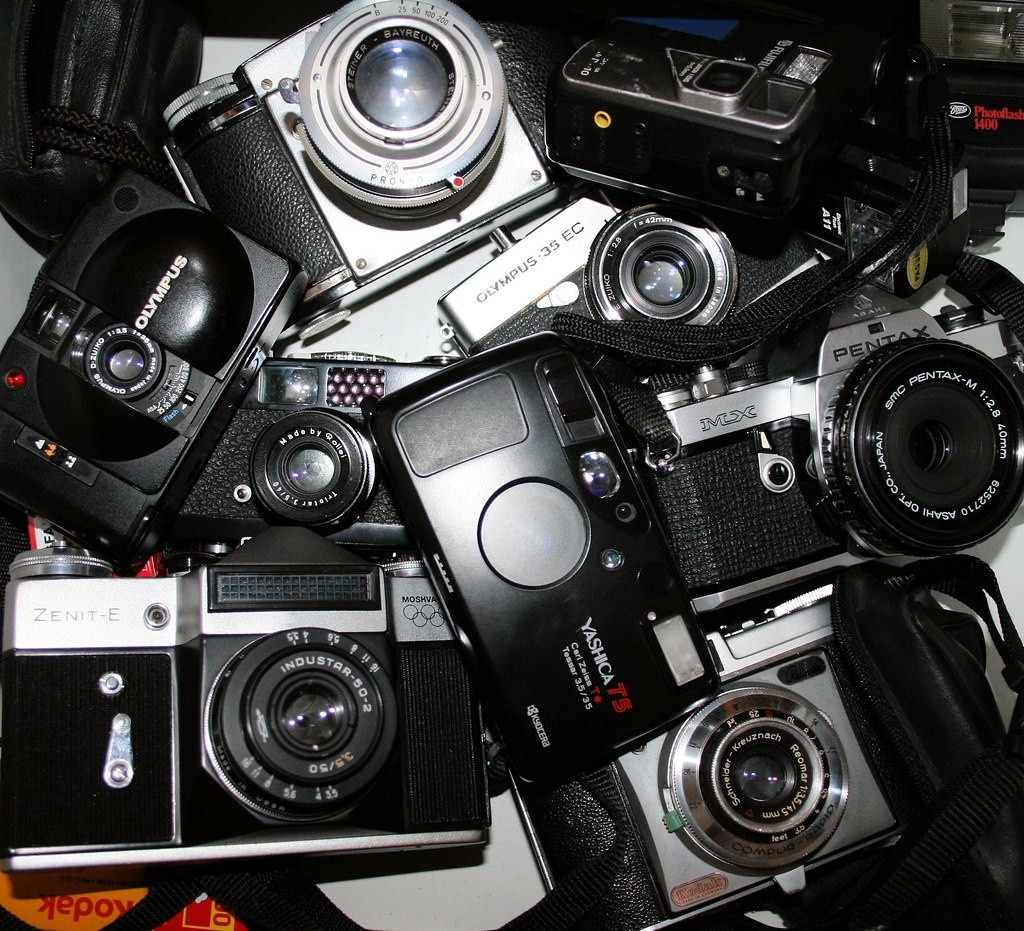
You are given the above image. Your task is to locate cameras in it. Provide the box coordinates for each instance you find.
[0,0,1024,931]
